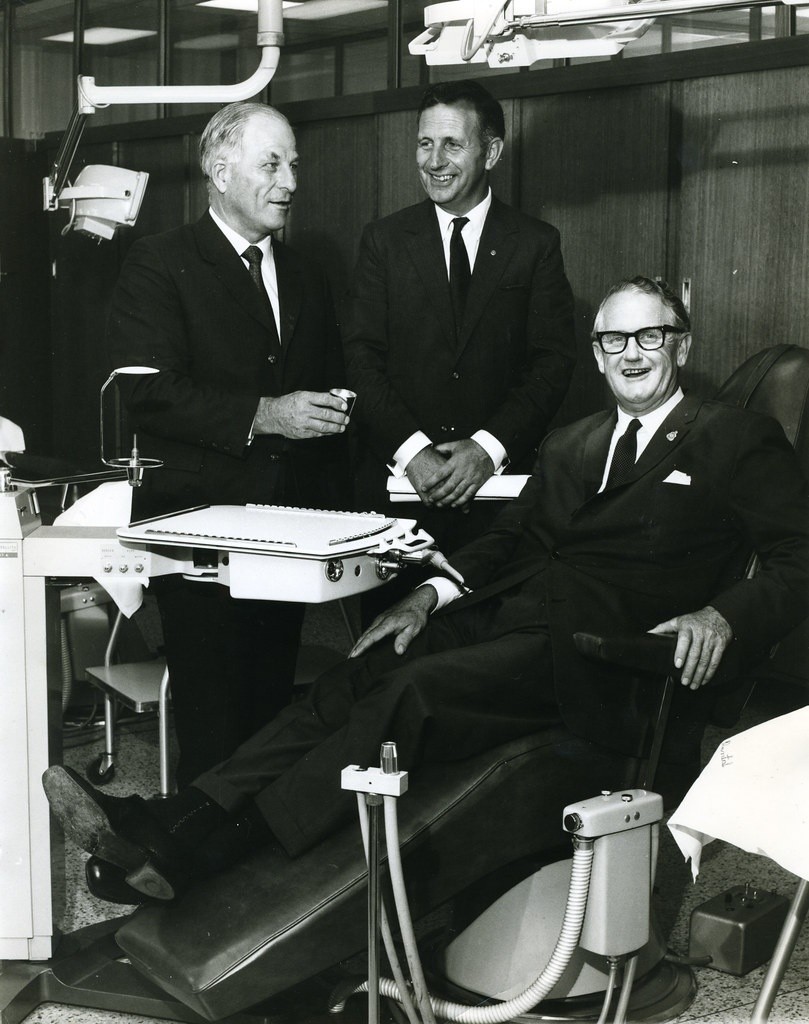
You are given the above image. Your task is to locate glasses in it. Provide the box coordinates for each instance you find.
[596,324,683,355]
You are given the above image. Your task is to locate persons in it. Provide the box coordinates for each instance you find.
[39,274,809,908]
[338,78,578,635]
[103,101,350,870]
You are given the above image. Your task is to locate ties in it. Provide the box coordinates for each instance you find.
[241,245,281,349]
[603,419,644,493]
[449,217,472,347]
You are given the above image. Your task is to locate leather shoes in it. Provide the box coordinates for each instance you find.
[41,763,189,903]
[85,854,188,906]
